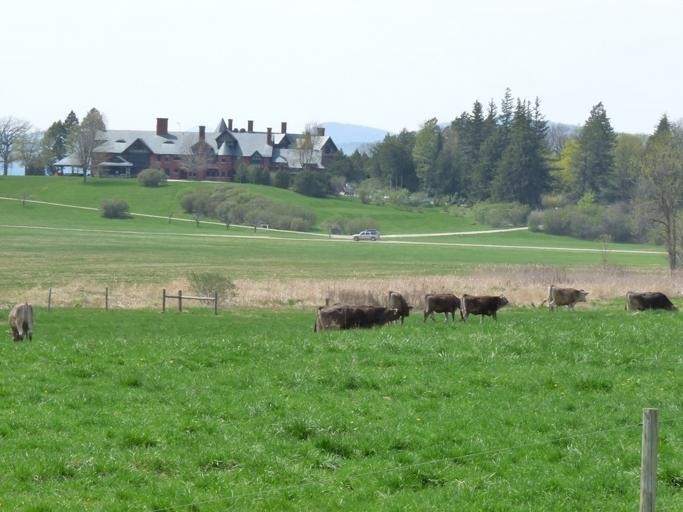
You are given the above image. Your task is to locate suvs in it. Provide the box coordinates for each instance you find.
[351,228,380,241]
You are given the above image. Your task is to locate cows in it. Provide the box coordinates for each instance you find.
[422,292,462,323]
[624,290,680,313]
[314,302,400,335]
[461,293,509,324]
[547,284,590,313]
[383,290,414,325]
[8,301,34,342]
